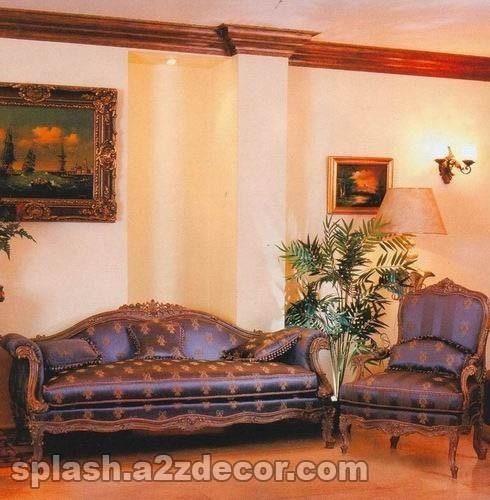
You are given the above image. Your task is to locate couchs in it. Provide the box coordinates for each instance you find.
[1,299,338,474]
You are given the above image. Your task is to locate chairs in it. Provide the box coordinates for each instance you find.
[338,276,489,480]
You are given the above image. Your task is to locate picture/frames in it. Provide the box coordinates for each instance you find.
[0,81,117,224]
[327,155,394,216]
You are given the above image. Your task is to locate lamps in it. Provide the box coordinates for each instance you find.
[372,188,448,302]
[434,140,476,184]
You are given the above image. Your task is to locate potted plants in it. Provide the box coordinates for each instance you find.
[274,215,418,439]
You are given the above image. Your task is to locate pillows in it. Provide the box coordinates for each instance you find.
[127,320,189,360]
[220,331,302,362]
[385,335,471,379]
[36,337,103,372]
